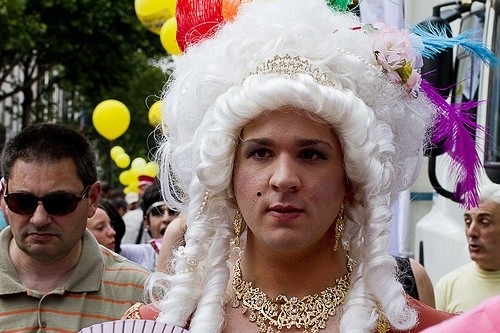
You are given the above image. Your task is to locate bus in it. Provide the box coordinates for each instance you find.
[395,0,500,261]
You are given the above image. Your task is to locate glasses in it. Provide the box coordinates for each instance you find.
[4,182,93,215]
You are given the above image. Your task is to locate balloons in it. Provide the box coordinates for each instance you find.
[94,98,164,197]
[134,0,239,56]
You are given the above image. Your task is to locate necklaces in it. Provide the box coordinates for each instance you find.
[230,257,357,333]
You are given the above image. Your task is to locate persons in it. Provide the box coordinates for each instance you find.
[0,123,153,333]
[433,183,500,314]
[393,255,436,310]
[121,0,458,320]
[84,174,190,277]
